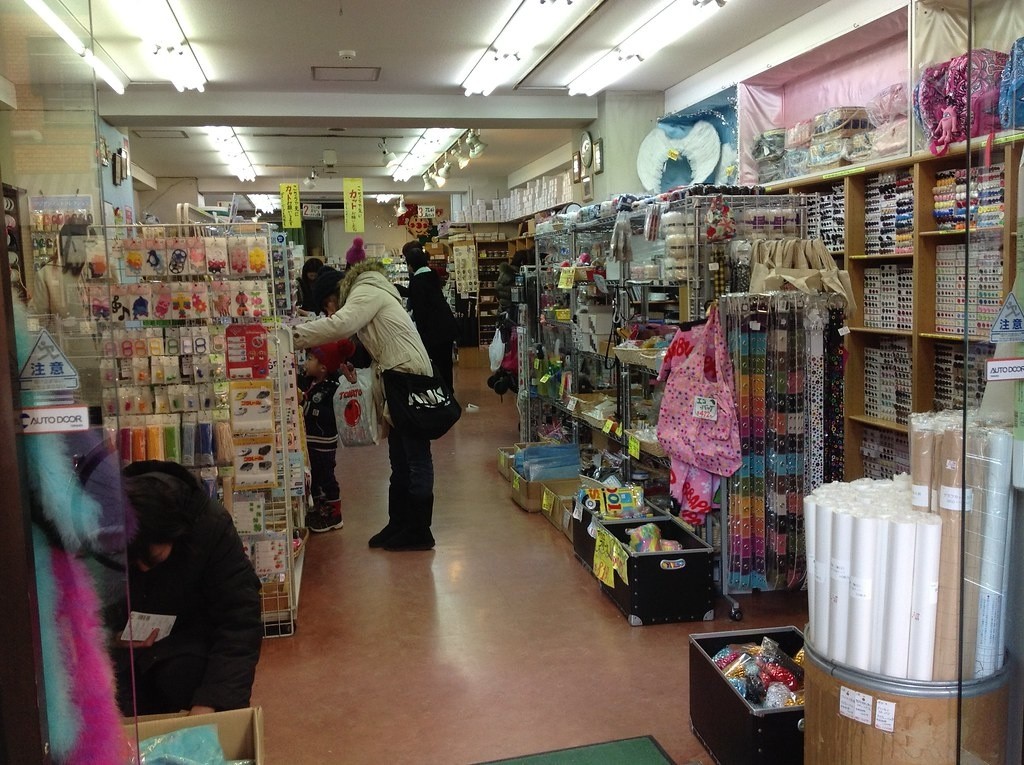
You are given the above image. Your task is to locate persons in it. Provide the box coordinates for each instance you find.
[99,460,263,716]
[395,241,424,322]
[406,248,456,397]
[296,337,355,533]
[341,237,365,276]
[495,249,534,353]
[296,258,435,552]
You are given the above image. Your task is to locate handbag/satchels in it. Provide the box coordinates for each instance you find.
[489,328,506,371]
[658,307,742,479]
[333,368,379,448]
[383,369,462,440]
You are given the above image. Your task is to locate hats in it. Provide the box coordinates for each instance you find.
[312,266,345,303]
[346,238,365,265]
[308,339,356,373]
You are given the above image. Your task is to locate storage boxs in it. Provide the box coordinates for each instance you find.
[123,704,264,765]
[686,625,806,765]
[452,173,572,222]
[499,443,717,626]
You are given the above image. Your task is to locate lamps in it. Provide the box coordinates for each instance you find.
[392,127,488,190]
[457,1,728,96]
[23,1,275,223]
[397,195,407,213]
[303,170,319,189]
[377,143,397,168]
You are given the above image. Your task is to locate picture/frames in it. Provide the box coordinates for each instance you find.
[114,152,122,186]
[581,173,594,201]
[593,137,604,174]
[572,151,581,183]
[580,131,593,168]
[120,148,128,180]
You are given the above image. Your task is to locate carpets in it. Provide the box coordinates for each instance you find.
[471,734,675,765]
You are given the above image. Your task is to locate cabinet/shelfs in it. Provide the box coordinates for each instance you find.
[741,130,1024,492]
[436,230,474,316]
[534,194,806,502]
[471,240,507,349]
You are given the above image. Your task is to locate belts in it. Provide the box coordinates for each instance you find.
[727,291,848,591]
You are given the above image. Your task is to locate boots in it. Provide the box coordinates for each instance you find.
[309,497,344,532]
[305,495,324,526]
[384,493,436,551]
[369,486,403,548]
